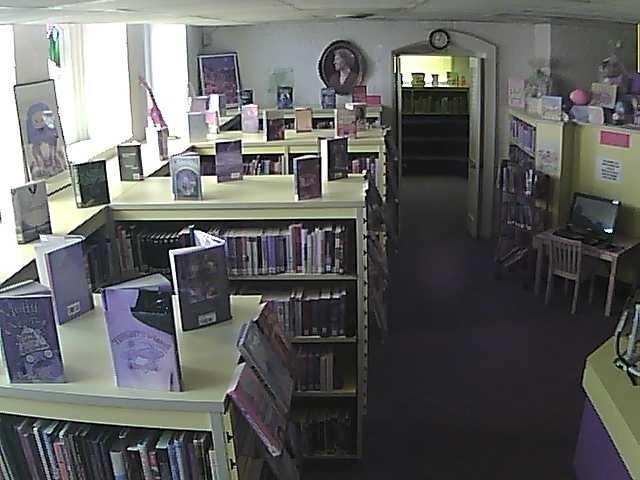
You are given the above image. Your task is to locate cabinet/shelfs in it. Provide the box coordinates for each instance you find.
[507,108,564,178]
[2,286,268,476]
[401,83,470,176]
[184,112,390,363]
[108,168,377,463]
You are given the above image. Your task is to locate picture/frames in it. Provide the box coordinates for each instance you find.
[315,38,365,97]
[13,78,73,196]
[197,51,241,110]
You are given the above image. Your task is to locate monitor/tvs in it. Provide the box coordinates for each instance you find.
[566,192,621,242]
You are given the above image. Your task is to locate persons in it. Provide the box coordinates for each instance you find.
[327,49,358,90]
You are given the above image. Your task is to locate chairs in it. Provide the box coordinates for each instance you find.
[544,232,597,315]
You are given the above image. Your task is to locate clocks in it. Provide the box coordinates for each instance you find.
[429,29,450,51]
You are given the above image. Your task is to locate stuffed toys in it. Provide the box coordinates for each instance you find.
[561,39,640,129]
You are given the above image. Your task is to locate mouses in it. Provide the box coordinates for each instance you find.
[607,243,616,249]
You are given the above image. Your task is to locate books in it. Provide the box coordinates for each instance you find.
[189,95,210,112]
[36,234,95,325]
[214,139,243,183]
[238,88,255,111]
[493,75,563,281]
[264,110,285,141]
[336,110,357,139]
[10,179,52,244]
[242,104,259,132]
[170,230,232,331]
[71,159,111,208]
[276,85,294,110]
[293,155,322,200]
[210,93,228,118]
[260,120,380,129]
[0,280,66,383]
[320,87,336,110]
[348,153,379,185]
[171,152,202,200]
[352,85,366,102]
[158,127,168,160]
[101,274,184,391]
[200,156,216,175]
[207,111,220,133]
[366,95,382,105]
[403,94,469,114]
[117,141,144,181]
[187,114,209,143]
[117,222,351,275]
[87,239,114,289]
[228,283,358,480]
[242,154,284,175]
[295,108,312,132]
[321,136,348,182]
[0,414,219,480]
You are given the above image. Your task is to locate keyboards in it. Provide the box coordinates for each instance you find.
[552,228,605,246]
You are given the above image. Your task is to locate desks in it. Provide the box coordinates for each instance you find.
[534,222,640,316]
